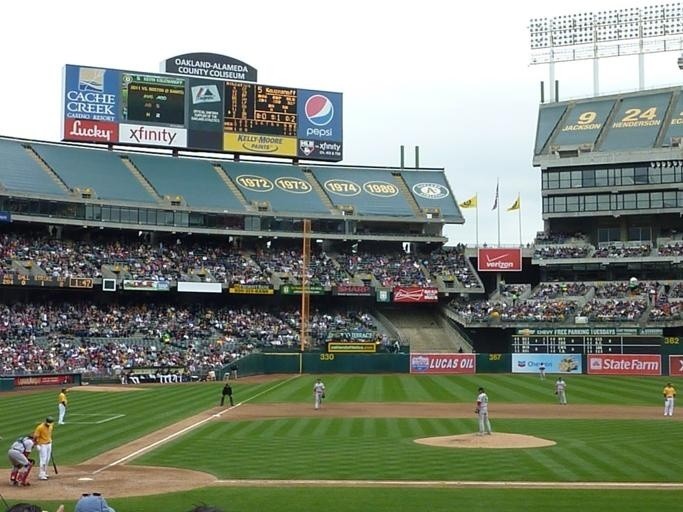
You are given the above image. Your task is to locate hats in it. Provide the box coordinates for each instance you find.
[74,493,116,512]
[666,382,674,387]
[45,415,54,423]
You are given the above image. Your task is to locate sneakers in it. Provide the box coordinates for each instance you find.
[38,473,49,480]
[8,479,30,487]
[663,410,675,418]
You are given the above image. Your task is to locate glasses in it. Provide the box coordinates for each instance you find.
[81,492,100,496]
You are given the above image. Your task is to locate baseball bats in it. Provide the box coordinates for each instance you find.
[50,452,57,474]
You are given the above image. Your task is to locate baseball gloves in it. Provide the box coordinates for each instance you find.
[475,407,480,413]
[26,459,35,467]
[665,397,668,401]
[322,392,325,399]
[555,391,559,395]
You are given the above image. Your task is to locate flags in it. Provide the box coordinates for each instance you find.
[460,195,477,208]
[492,185,498,210]
[506,195,521,211]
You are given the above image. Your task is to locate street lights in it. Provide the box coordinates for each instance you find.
[531,3,683,102]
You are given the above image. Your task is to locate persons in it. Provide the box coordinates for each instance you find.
[527,233,683,260]
[9,500,64,511]
[1,233,478,294]
[7,435,36,486]
[663,381,677,416]
[33,416,55,480]
[539,363,546,380]
[556,377,568,405]
[448,282,683,327]
[220,383,234,407]
[476,387,492,437]
[57,388,67,424]
[313,378,324,409]
[458,346,463,353]
[1,302,400,386]
